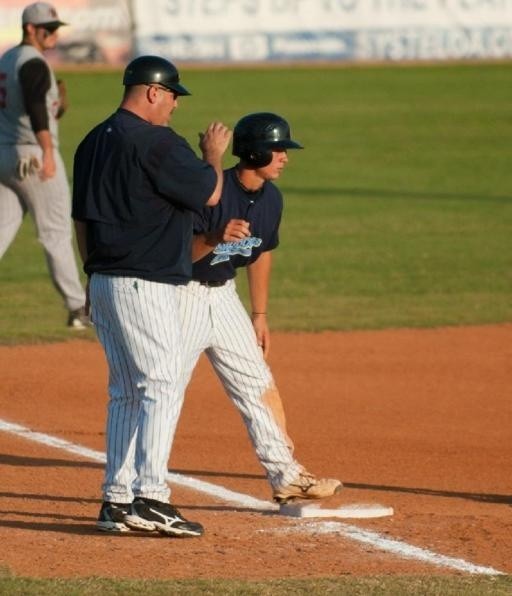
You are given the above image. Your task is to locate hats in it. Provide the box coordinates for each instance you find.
[22,3,69,29]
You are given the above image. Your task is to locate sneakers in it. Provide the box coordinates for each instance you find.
[272,470,343,504]
[96,497,132,535]
[124,496,203,535]
[67,307,95,329]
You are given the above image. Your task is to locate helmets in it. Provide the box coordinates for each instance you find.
[232,112,304,169]
[123,54,191,96]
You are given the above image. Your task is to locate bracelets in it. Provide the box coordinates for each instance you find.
[253,311,269,316]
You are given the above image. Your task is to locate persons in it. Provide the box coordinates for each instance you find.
[73,54,235,535]
[173,111,344,508]
[0,2,98,328]
